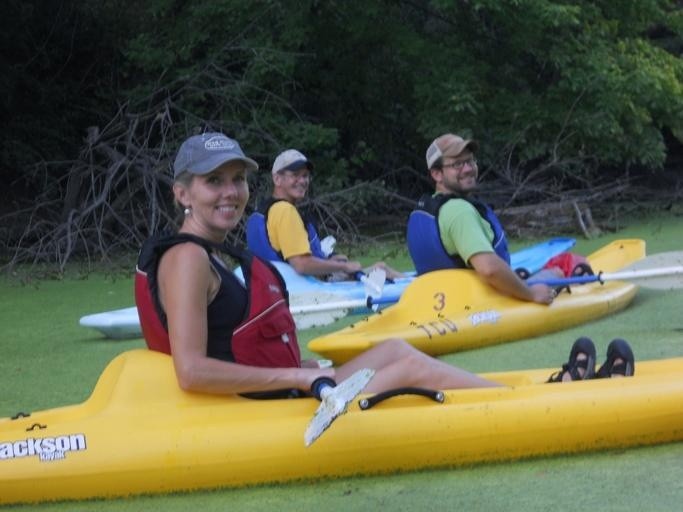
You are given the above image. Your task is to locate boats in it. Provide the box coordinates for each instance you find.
[304,236,649,362]
[74,238,576,338]
[0,353,682,508]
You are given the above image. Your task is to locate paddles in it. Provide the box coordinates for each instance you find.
[305,359,374,445]
[320,235,388,300]
[289,251,683,330]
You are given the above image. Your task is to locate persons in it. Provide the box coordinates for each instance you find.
[133,130,635,402]
[245,147,409,285]
[403,132,567,308]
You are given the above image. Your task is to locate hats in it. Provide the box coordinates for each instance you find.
[271,149,314,174]
[173,131,258,181]
[426,132,480,170]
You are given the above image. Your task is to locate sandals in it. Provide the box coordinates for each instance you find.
[546,337,596,382]
[596,338,634,377]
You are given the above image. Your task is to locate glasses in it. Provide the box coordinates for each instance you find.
[442,158,475,169]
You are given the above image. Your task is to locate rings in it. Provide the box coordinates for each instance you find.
[550,288,555,294]
